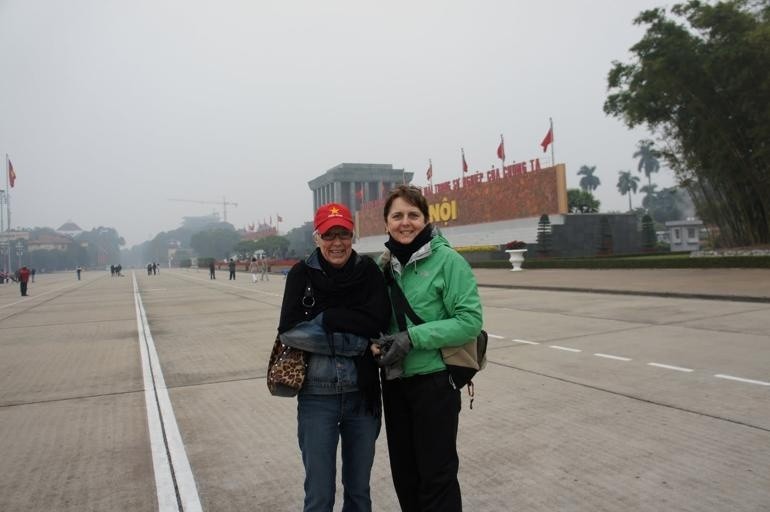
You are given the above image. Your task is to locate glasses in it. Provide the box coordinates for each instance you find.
[315,231,353,242]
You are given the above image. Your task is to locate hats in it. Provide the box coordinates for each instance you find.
[314,203,355,235]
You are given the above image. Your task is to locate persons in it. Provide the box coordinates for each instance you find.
[373,181,485,512]
[110,263,122,278]
[277,202,387,512]
[207,259,216,279]
[249,257,261,282]
[0,268,36,283]
[18,266,29,296]
[229,259,236,281]
[146,262,161,277]
[259,255,269,281]
[77,266,82,281]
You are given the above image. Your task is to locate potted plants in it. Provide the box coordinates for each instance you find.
[503,239,529,272]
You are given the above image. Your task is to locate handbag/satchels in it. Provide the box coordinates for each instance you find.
[437,326,490,391]
[266,314,315,398]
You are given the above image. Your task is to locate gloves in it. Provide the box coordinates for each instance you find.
[374,330,412,370]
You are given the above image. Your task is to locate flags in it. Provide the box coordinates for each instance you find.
[379,179,385,195]
[402,170,410,186]
[9,160,17,189]
[498,140,506,162]
[541,124,554,154]
[426,163,432,182]
[243,213,283,231]
[356,190,365,201]
[462,153,468,173]
